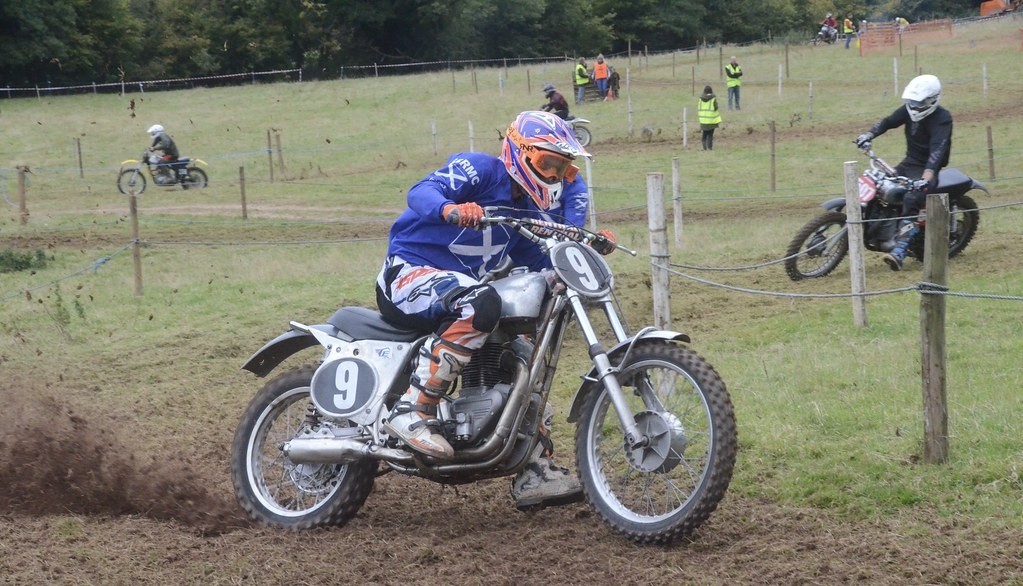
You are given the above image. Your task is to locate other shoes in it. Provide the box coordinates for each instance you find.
[883,254,903,269]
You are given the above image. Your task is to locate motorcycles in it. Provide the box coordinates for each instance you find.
[539,104,592,147]
[117,146,208,195]
[783,140,990,281]
[229,214,738,548]
[814,23,837,46]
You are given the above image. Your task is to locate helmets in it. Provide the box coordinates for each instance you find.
[544,85,555,98]
[503,110,592,212]
[901,74,941,121]
[148,124,164,138]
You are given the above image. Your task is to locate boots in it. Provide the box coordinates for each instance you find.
[383,334,475,461]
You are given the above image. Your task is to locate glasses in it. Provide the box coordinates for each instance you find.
[909,96,935,110]
[530,150,573,182]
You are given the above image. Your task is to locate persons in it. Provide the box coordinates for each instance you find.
[542,83,569,120]
[148,125,179,180]
[894,16,909,35]
[591,53,610,96]
[605,67,620,97]
[373,109,592,515]
[725,55,743,111]
[821,11,839,29]
[699,86,722,151]
[576,57,591,104]
[856,74,953,271]
[844,12,855,49]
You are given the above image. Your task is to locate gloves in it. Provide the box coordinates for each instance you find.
[443,200,487,232]
[856,134,872,148]
[919,179,930,192]
[587,228,617,253]
